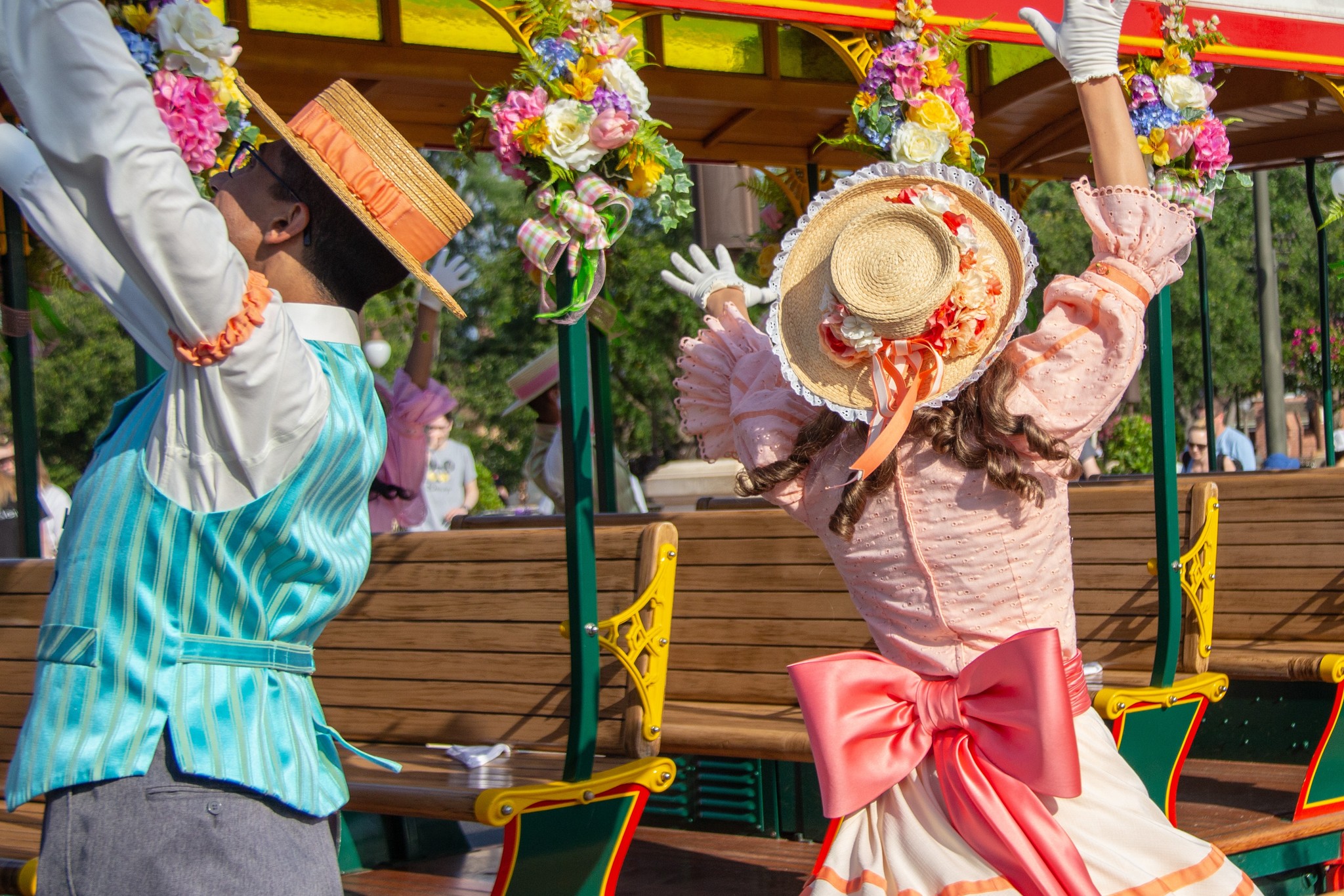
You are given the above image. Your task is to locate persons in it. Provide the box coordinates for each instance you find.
[502,345,649,514]
[661,0,1265,896]
[0,1,475,896]
[369,248,478,533]
[0,421,71,558]
[1070,395,1344,482]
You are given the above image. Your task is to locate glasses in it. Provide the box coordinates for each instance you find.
[228,140,313,248]
[1187,443,1207,451]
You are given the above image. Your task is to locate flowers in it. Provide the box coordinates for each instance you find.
[1080,0,1256,198]
[20,0,280,303]
[444,0,697,238]
[811,184,1006,371]
[811,0,999,194]
[1285,311,1344,392]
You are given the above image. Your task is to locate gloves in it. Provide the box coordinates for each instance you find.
[1018,0,1131,85]
[659,243,776,315]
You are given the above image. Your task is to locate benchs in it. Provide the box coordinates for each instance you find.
[0,466,1344,896]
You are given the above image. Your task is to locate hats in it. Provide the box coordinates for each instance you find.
[232,75,475,322]
[767,163,1041,493]
[502,344,559,418]
